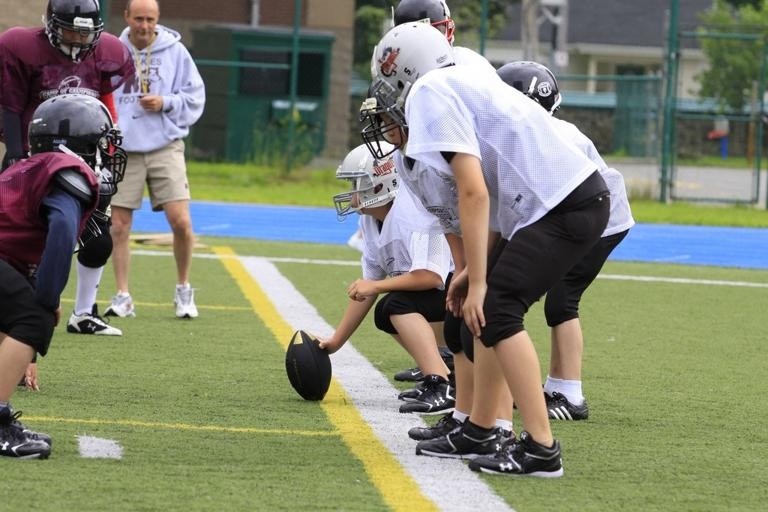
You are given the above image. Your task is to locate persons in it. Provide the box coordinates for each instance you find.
[0,94,130,460]
[359,78,516,450]
[494,62,636,420]
[103,0,206,318]
[761,111,768,157]
[305,141,456,414]
[0,0,139,336]
[371,21,611,478]
[393,0,456,382]
[714,105,729,160]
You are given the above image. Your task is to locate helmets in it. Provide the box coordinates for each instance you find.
[28,96,127,197]
[497,62,562,117]
[394,1,455,46]
[46,0,104,61]
[332,142,404,214]
[361,82,409,158]
[371,21,454,111]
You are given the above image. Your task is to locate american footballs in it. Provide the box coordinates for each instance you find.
[285,331,332,401]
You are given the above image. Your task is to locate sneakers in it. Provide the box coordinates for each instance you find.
[397,379,438,402]
[408,413,463,440]
[400,375,457,414]
[512,385,559,410]
[0,409,50,460]
[104,295,133,316]
[469,432,563,478]
[415,421,500,460]
[395,366,422,381]
[67,305,122,336]
[546,393,589,421]
[10,409,51,447]
[440,355,455,370]
[174,285,198,318]
[496,427,515,445]
